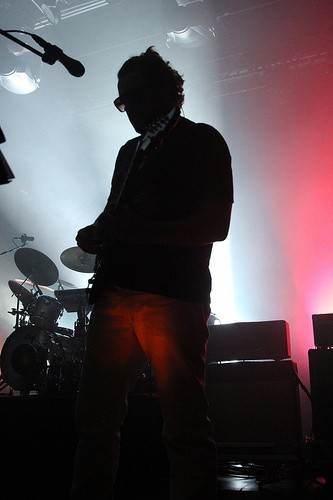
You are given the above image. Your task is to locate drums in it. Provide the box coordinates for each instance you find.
[30,295,64,327]
[0,325,81,392]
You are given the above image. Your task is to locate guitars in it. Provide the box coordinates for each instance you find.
[84,107,182,307]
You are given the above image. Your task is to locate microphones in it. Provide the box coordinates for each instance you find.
[32,34,85,77]
[36,284,43,295]
[16,234,34,241]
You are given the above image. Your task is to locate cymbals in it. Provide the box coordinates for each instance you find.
[54,279,77,288]
[8,280,37,306]
[15,279,54,292]
[61,247,97,273]
[14,248,59,287]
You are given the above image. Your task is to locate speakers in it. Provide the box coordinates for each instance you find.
[205,359,301,454]
[308,348,333,453]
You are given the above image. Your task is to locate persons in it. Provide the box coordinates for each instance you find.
[69,46,233,500]
